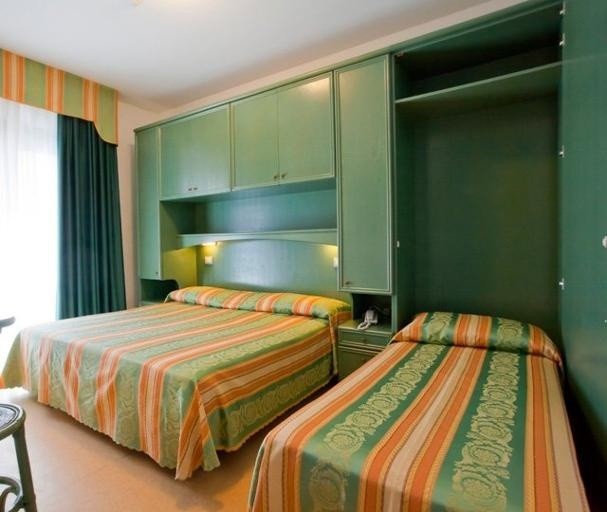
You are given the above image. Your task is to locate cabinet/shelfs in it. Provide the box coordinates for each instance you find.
[133,120,161,280]
[158,96,232,202]
[230,66,335,191]
[333,0,607,333]
[337,320,393,383]
[138,299,164,308]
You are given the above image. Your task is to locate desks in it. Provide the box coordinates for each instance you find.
[0,314,16,336]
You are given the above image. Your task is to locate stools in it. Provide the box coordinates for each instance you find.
[1,403,38,512]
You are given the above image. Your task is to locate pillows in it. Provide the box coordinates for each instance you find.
[164,285,351,375]
[390,311,565,390]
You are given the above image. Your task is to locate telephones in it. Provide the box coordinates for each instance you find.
[365,310,378,324]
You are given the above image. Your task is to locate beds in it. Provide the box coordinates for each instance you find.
[248,312,590,512]
[0,285,351,481]
